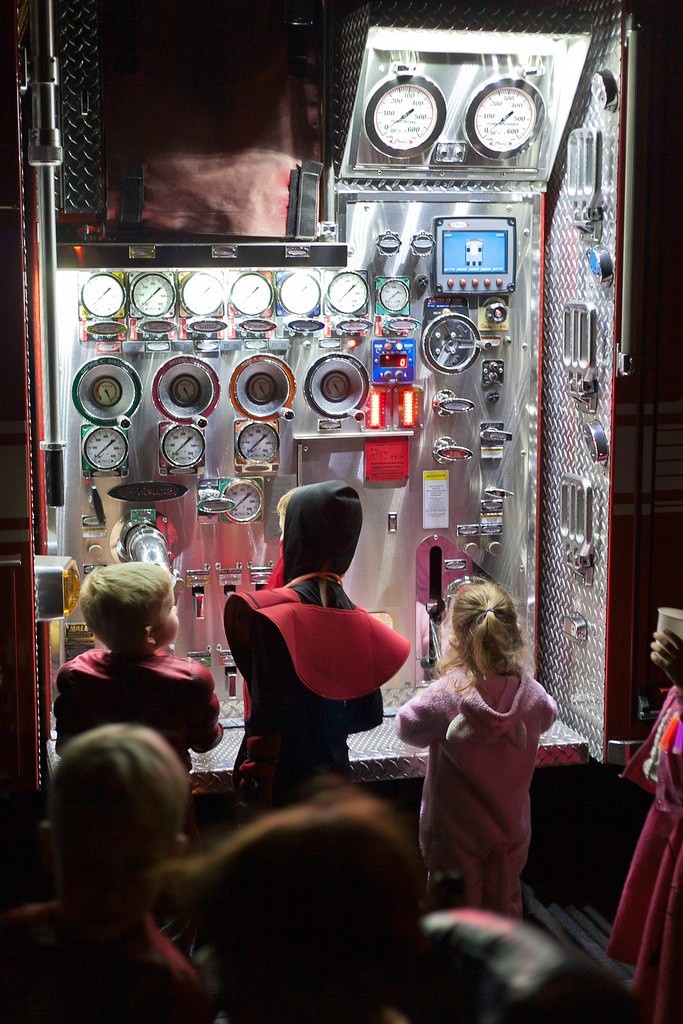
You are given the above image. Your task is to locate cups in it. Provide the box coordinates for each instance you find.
[656,607,683,657]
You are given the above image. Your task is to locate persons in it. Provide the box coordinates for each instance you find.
[223,480,410,819]
[181,787,423,1024]
[391,583,561,926]
[607,626,683,1024]
[54,560,223,772]
[0,726,201,1024]
